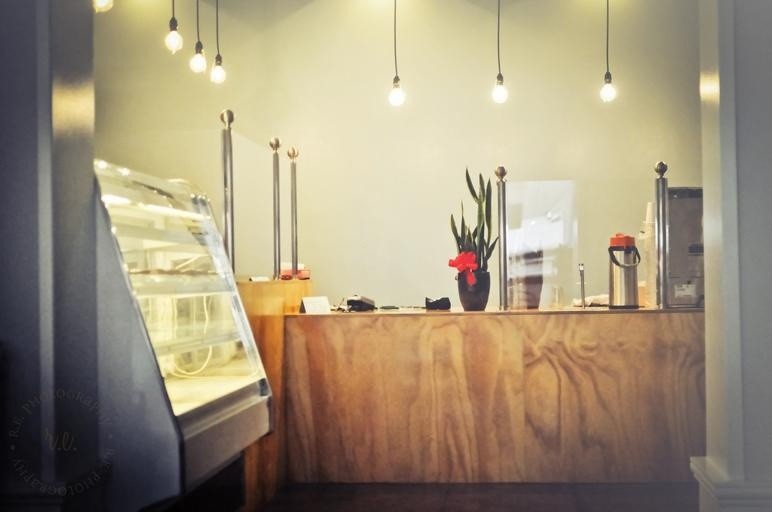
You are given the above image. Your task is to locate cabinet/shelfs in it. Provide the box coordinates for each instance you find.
[93,158,273,511]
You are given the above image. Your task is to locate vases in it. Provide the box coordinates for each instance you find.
[457,270,491,312]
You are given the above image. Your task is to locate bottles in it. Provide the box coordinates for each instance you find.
[608,234,641,308]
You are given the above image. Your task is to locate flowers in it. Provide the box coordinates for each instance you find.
[449,165,499,286]
